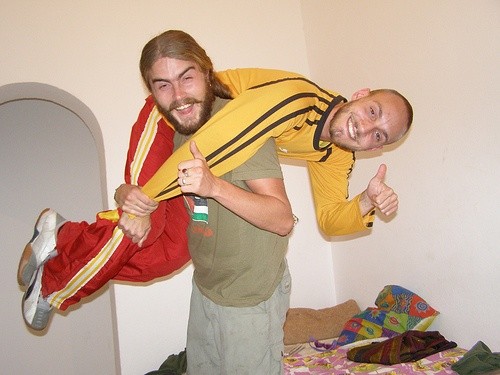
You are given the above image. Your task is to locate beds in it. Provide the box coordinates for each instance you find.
[281,334,468,375]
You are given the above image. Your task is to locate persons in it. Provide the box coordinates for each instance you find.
[114,30,295,375]
[17,68,413,331]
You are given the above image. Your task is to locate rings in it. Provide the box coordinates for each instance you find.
[182,177,187,185]
[182,169,189,177]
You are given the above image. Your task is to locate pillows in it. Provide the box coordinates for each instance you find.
[284,300,362,344]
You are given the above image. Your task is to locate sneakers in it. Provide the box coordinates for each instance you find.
[17,208,68,285]
[22,256,54,331]
